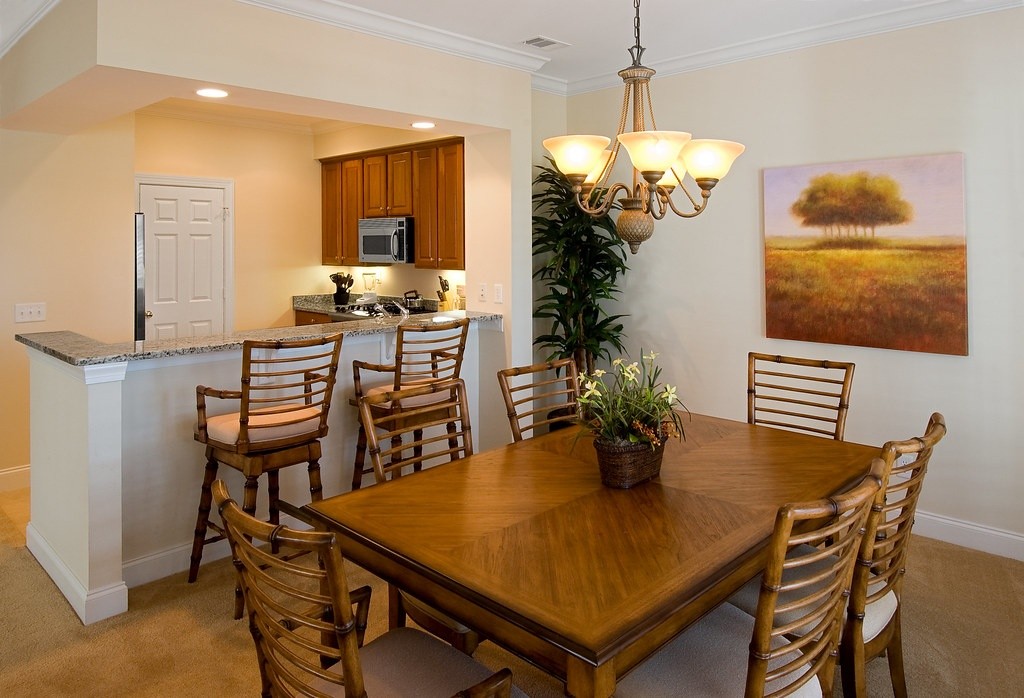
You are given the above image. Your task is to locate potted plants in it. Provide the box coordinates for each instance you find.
[532,155,632,433]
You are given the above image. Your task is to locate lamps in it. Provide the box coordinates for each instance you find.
[543,0,745,254]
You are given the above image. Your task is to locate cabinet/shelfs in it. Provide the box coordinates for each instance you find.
[412,142,465,270]
[321,158,364,266]
[295,310,332,327]
[363,151,412,218]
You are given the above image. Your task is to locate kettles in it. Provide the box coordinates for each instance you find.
[403,290,423,308]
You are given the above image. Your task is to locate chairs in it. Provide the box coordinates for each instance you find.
[497,358,584,443]
[188,332,344,620]
[727,412,947,697]
[748,351,856,557]
[616,459,887,698]
[358,378,488,656]
[212,478,528,698]
[349,317,473,491]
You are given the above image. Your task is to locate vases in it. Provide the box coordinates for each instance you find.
[593,435,668,489]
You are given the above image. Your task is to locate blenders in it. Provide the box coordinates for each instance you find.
[356,273,377,304]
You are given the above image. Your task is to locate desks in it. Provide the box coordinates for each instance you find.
[300,409,901,698]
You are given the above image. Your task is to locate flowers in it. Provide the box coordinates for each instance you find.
[569,347,691,456]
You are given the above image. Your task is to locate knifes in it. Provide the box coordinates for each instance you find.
[436,276,449,301]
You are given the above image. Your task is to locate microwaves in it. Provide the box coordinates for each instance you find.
[358,217,415,264]
